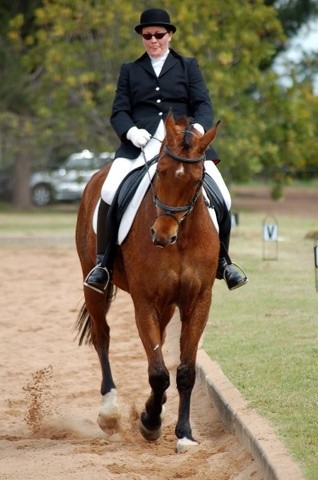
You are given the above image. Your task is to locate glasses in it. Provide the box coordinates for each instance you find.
[141,31,168,40]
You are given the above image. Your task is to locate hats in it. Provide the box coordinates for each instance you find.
[135,9,176,34]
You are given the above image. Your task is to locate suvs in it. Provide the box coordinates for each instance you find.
[28,149,116,207]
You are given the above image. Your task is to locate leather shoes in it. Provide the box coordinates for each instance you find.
[216,257,245,287]
[88,254,113,283]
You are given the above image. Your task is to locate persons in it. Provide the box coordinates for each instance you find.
[83,8,248,295]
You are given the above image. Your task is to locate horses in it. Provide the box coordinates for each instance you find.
[72,106,221,453]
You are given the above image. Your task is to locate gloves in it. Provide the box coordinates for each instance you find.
[126,126,152,148]
[192,123,204,135]
